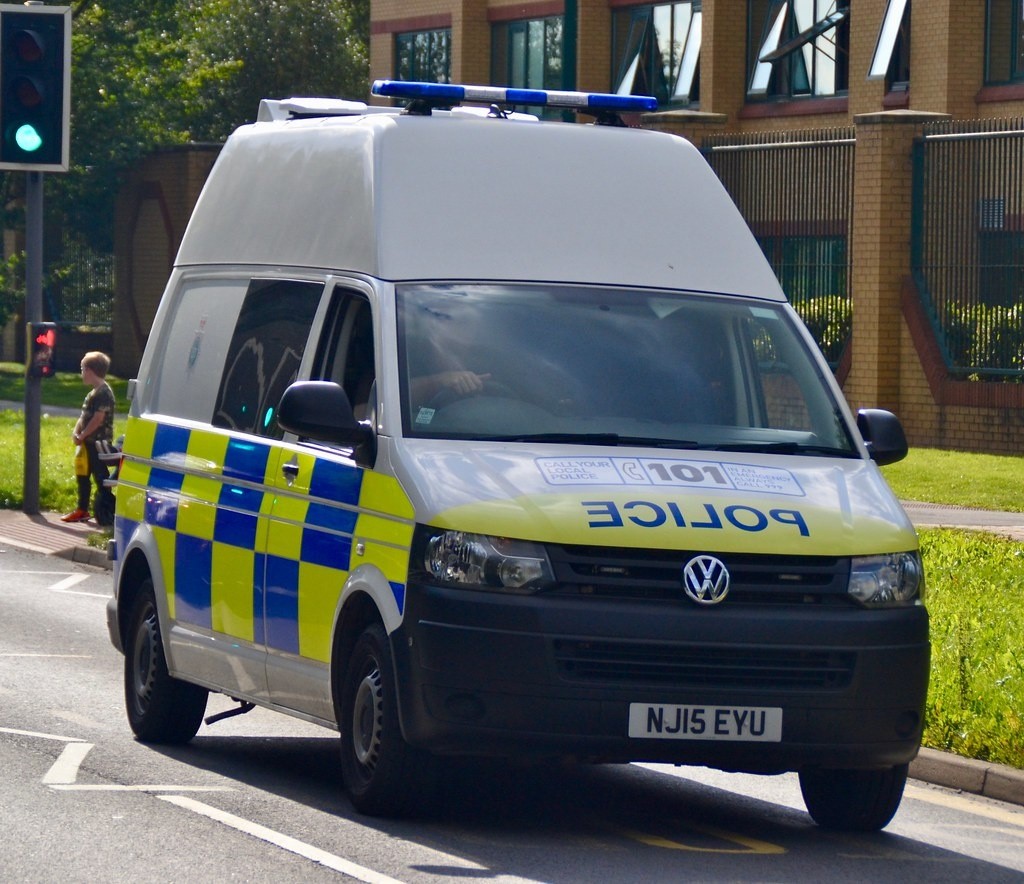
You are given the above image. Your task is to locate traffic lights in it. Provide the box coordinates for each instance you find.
[0,4,71,171]
[25,321,58,379]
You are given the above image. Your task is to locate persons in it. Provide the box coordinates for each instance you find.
[371,303,501,418]
[60,352,117,525]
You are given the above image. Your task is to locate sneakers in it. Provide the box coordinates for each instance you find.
[61,509,91,522]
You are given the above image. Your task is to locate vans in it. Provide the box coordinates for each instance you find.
[103,80,930,833]
